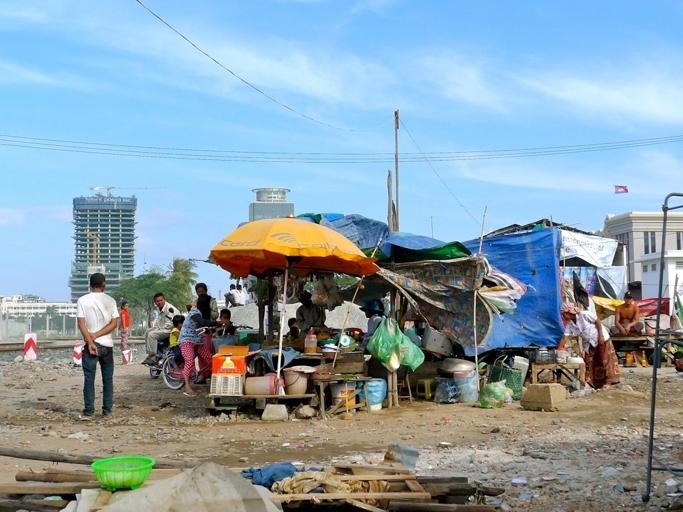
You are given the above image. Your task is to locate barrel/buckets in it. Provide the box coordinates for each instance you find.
[245,377,276,395]
[330,382,356,413]
[644,317,660,335]
[285,373,307,394]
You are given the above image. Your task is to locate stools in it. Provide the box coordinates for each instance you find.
[417,379,436,401]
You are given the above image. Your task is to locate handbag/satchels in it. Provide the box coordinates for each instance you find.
[488,355,521,400]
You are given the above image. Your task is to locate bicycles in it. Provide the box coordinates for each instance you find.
[141,323,253,389]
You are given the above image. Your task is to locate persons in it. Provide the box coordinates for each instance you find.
[189,282,219,336]
[119,300,130,350]
[608,290,644,335]
[285,317,299,337]
[75,272,119,418]
[139,291,179,364]
[229,285,245,306]
[224,284,240,308]
[289,326,300,340]
[556,301,620,387]
[212,308,238,344]
[295,291,327,333]
[167,314,183,368]
[177,293,228,398]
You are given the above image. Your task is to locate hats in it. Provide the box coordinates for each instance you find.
[625,291,632,298]
[359,299,384,312]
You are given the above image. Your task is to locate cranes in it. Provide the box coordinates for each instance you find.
[90,184,153,198]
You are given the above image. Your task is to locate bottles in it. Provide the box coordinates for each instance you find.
[304,334,317,353]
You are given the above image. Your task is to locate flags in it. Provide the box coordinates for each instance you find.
[614,185,628,194]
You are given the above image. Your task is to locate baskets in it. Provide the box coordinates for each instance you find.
[91,456,155,491]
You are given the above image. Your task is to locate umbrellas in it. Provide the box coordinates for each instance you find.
[204,212,381,393]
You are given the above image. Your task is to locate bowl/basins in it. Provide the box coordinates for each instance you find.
[439,358,477,375]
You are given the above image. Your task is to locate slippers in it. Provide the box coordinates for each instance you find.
[142,355,153,364]
[182,391,196,397]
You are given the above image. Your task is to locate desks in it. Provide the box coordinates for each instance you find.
[609,331,675,368]
[531,356,586,392]
